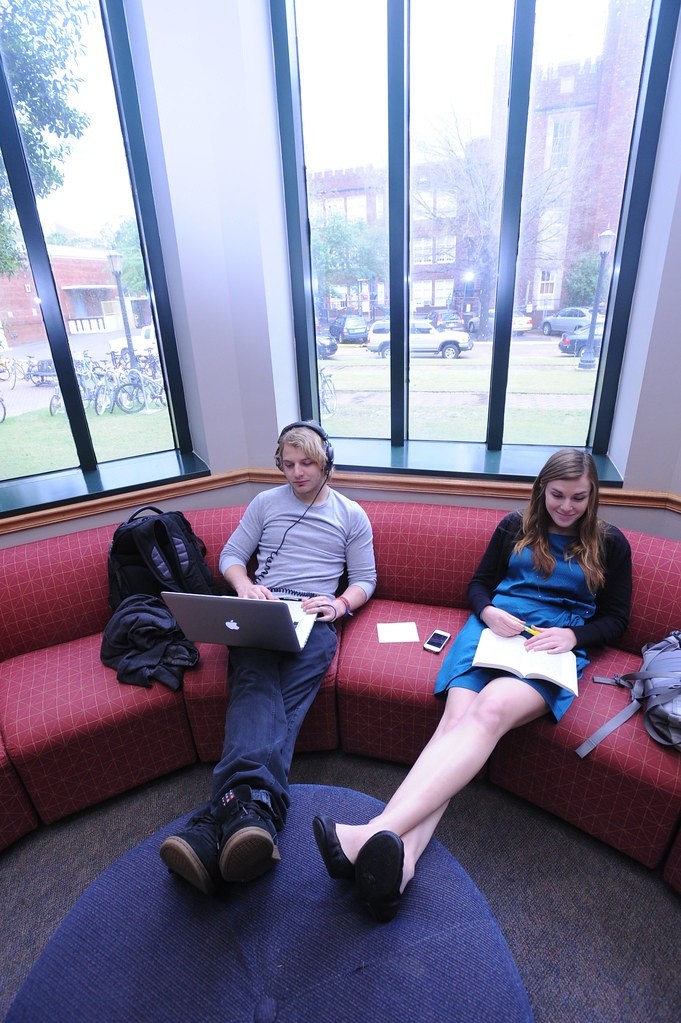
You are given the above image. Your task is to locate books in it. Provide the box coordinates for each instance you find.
[473,626,578,697]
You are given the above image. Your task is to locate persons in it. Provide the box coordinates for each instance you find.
[312,448,632,925]
[160,421,377,893]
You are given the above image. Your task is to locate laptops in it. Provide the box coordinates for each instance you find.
[161,590,318,652]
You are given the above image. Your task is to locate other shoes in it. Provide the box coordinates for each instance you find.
[354,830,404,924]
[313,815,358,879]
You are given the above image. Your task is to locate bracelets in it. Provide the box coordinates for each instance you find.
[337,597,353,618]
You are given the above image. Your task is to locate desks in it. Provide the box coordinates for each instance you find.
[7,783,532,1023]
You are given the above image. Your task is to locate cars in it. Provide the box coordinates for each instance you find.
[539,305,605,336]
[558,322,605,357]
[316,328,337,359]
[468,308,533,336]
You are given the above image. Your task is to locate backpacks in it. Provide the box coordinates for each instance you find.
[105,504,231,621]
[614,629,680,753]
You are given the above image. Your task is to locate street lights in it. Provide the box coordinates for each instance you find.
[105,249,140,370]
[577,220,617,369]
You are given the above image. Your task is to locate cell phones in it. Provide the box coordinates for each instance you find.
[423,630,451,654]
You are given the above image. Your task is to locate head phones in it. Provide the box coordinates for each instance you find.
[275,421,334,472]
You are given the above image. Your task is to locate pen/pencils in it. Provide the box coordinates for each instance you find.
[279,597,303,601]
[516,622,540,636]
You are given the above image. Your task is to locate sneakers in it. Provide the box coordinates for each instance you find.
[212,783,282,884]
[159,805,223,894]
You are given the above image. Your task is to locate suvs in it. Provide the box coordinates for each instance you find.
[425,309,466,333]
[330,312,370,345]
[367,318,473,358]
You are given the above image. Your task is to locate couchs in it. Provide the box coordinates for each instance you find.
[0,498,681,893]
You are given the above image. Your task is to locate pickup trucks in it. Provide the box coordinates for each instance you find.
[108,324,158,350]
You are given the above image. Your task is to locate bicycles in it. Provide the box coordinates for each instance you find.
[0,347,169,424]
[318,366,337,419]
